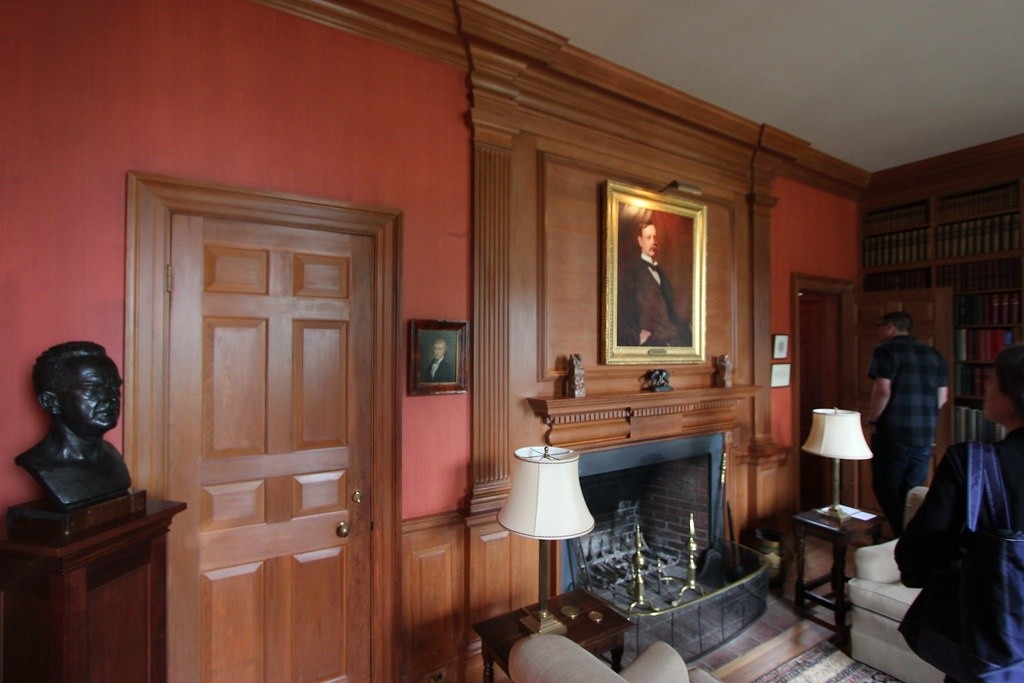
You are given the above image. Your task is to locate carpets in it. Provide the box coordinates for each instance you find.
[750,626,905,683]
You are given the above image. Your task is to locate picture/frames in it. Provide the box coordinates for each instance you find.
[407,318,470,396]
[773,334,789,360]
[600,179,708,366]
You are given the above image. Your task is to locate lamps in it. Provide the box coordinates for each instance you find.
[801,406,873,520]
[658,180,703,196]
[498,447,596,636]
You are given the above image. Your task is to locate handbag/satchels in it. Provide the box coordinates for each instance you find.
[897,441,1024,681]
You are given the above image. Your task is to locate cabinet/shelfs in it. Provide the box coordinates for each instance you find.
[860,184,1024,447]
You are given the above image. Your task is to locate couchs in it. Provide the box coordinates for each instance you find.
[847,486,946,683]
[508,632,723,683]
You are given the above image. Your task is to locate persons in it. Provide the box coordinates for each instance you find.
[423,336,455,383]
[16,342,131,508]
[896,345,1024,682]
[865,311,949,537]
[619,222,692,346]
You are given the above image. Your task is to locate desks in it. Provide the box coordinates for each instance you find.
[792,507,882,631]
[472,589,638,683]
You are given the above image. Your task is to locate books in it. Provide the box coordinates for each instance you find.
[861,180,1023,444]
